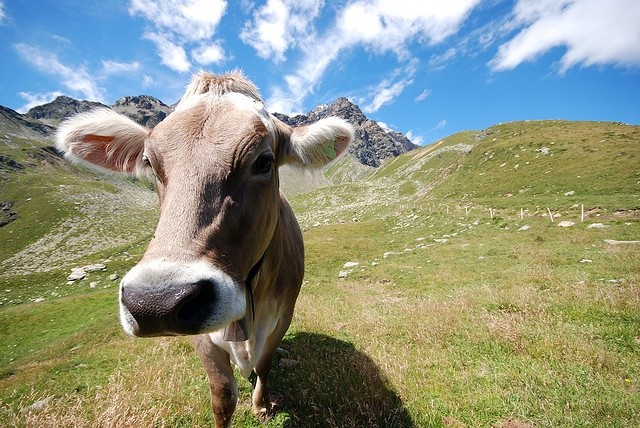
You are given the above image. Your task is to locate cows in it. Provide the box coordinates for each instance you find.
[50,65,356,428]
